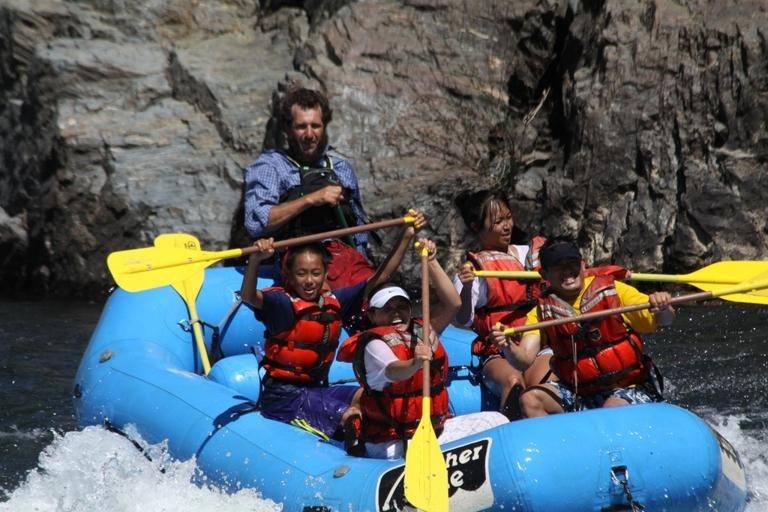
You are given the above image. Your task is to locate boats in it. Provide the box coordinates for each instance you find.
[76,261,752,511]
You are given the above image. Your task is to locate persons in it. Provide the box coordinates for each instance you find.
[450,189,549,420]
[242,208,429,440]
[335,237,510,459]
[489,234,676,418]
[243,89,374,268]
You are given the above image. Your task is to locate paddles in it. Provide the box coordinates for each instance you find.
[153,233,212,376]
[464,261,768,305]
[108,208,423,293]
[404,241,448,512]
[496,272,768,338]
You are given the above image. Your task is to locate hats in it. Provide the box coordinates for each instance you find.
[540,243,583,269]
[368,284,413,312]
